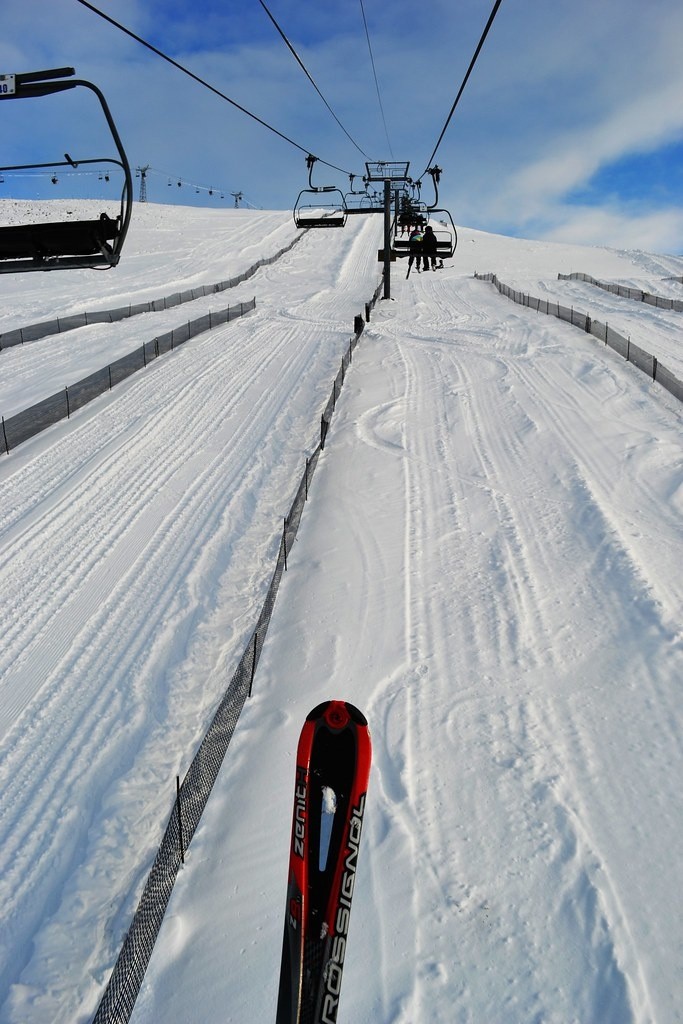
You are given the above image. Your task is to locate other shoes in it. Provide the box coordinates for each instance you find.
[423,267,429,271]
[432,266,436,271]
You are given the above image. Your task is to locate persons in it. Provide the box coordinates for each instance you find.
[398,211,423,238]
[423,226,437,271]
[406,230,423,279]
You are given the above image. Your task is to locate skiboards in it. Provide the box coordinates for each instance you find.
[274,701,372,1024]
